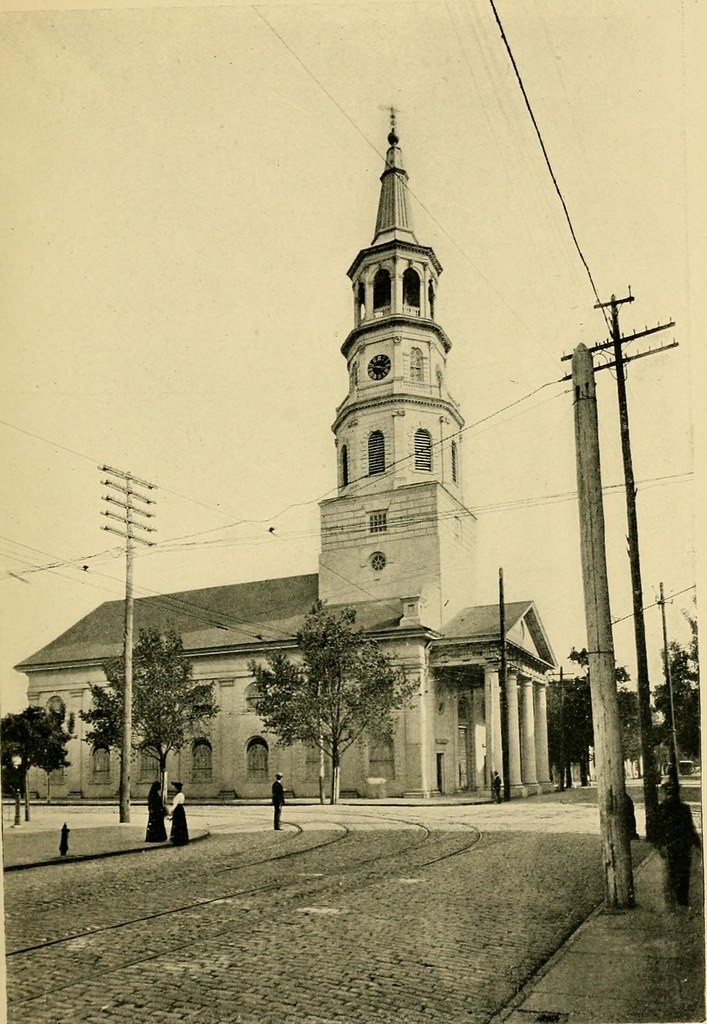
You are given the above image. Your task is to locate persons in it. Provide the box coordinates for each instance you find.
[145,781,169,842]
[493,771,501,803]
[169,782,189,845]
[646,782,700,906]
[272,773,286,830]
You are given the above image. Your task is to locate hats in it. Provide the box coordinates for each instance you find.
[171,781,183,787]
[662,782,681,793]
